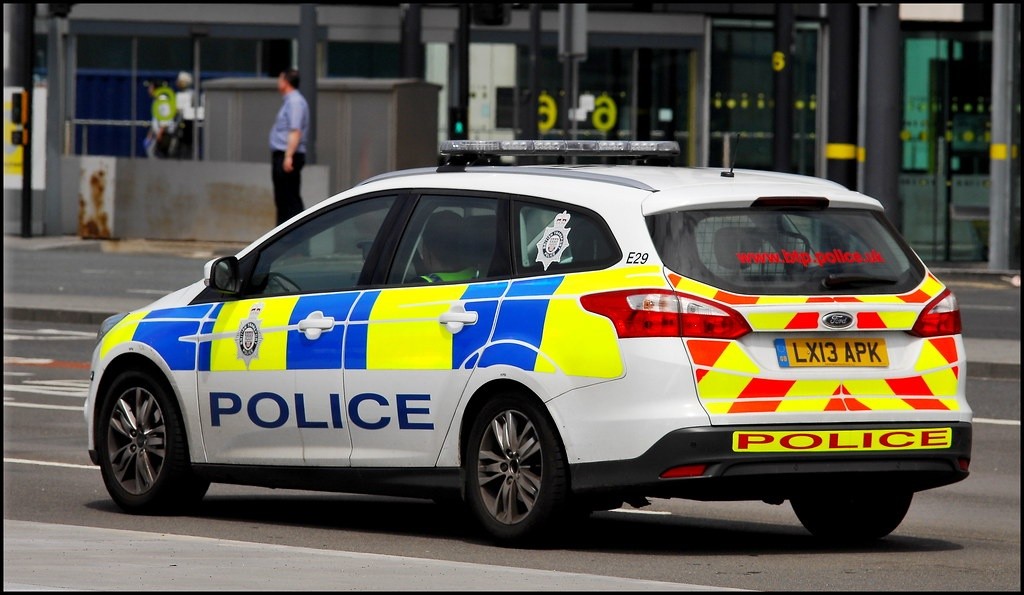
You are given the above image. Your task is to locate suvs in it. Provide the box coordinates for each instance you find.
[81,134,976,548]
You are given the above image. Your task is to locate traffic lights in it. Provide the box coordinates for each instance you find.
[447,107,469,141]
[10,91,28,146]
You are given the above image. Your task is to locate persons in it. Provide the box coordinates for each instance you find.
[143,76,184,160]
[268,69,310,226]
[175,70,205,160]
[409,210,480,284]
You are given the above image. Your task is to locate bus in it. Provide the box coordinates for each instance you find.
[948,90,1024,270]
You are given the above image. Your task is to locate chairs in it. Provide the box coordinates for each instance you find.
[713,227,760,282]
[814,233,860,276]
